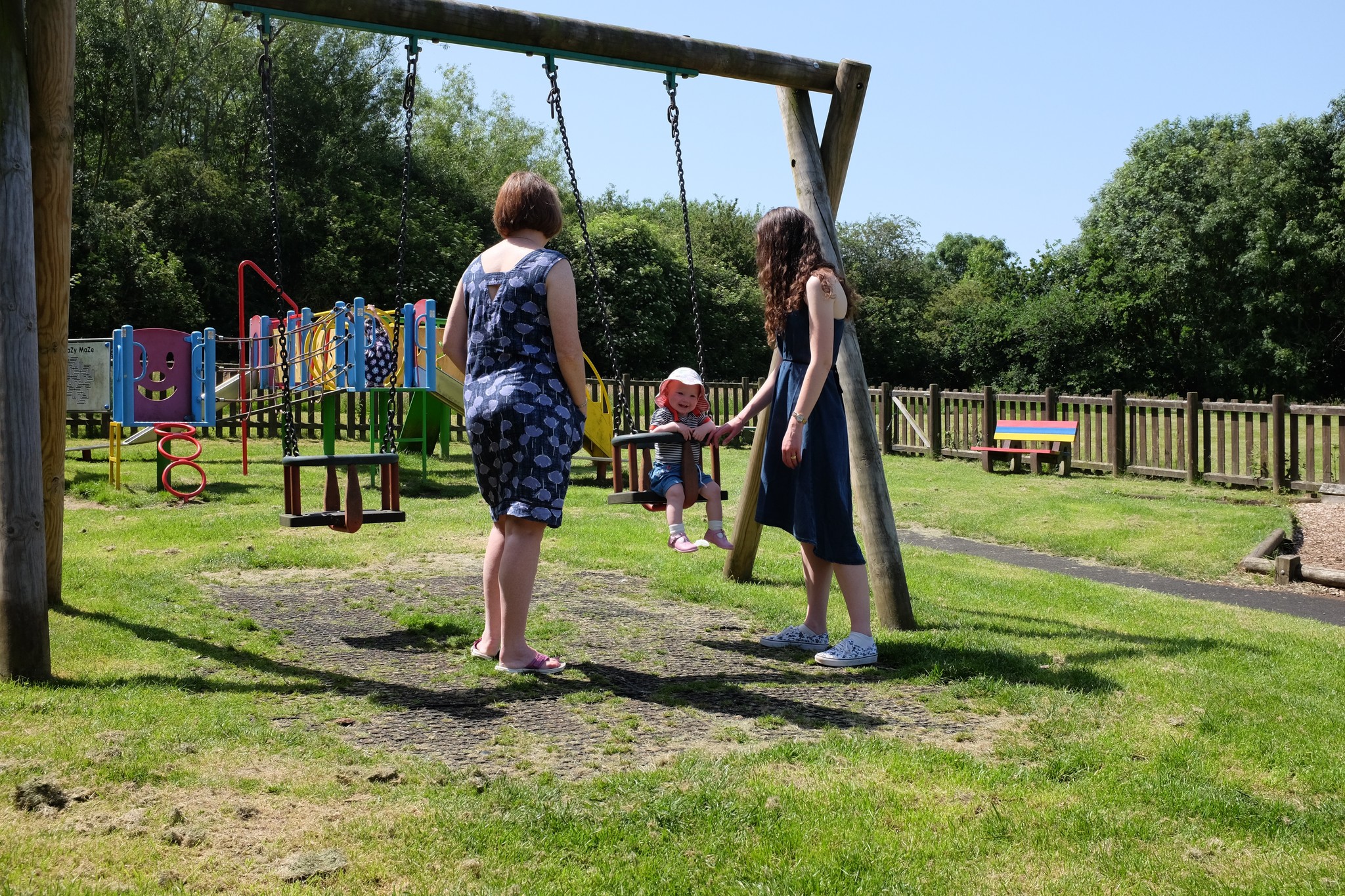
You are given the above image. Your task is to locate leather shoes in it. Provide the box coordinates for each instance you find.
[704,528,736,551]
[667,532,699,553]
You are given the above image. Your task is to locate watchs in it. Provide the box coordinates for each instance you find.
[792,411,807,424]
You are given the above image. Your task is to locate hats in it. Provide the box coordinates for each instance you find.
[654,367,710,413]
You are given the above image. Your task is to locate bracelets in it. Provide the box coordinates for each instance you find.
[576,398,587,408]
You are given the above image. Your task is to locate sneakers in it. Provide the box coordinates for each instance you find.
[815,637,879,666]
[760,625,829,651]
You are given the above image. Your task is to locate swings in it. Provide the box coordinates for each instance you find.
[254,7,423,533]
[542,54,729,512]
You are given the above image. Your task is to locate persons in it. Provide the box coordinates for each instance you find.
[650,367,733,553]
[705,206,879,668]
[442,173,588,673]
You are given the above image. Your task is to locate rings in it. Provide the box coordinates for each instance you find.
[791,456,796,459]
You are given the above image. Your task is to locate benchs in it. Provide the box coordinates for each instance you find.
[970,420,1079,477]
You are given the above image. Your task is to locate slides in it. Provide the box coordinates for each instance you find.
[123,366,259,446]
[396,391,442,455]
[429,366,465,417]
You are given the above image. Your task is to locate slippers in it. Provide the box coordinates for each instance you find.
[470,636,501,662]
[494,652,566,675]
